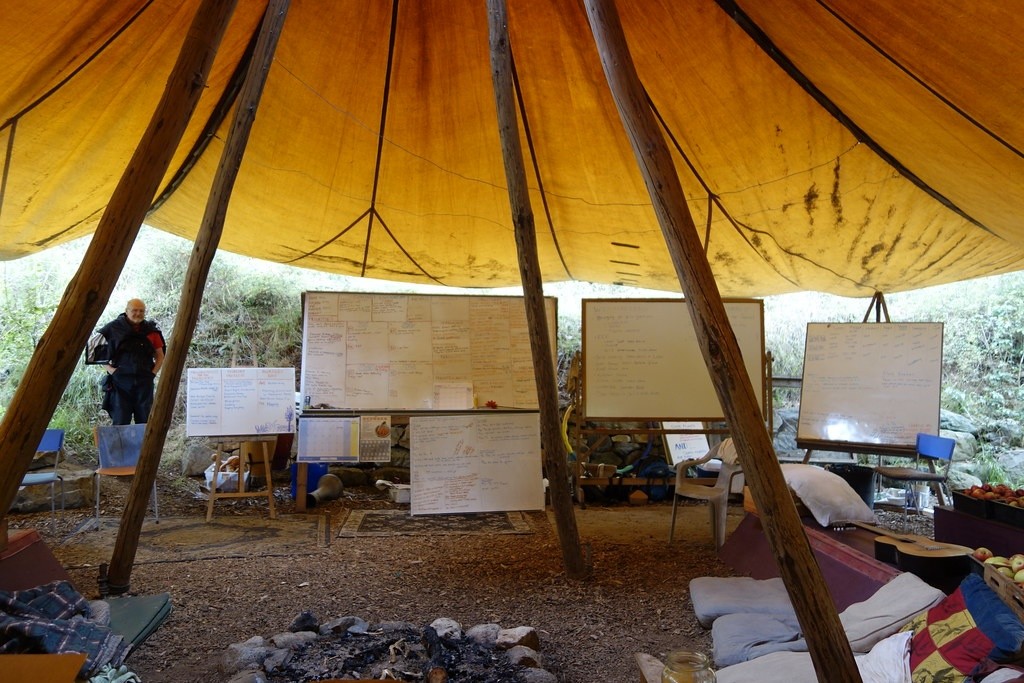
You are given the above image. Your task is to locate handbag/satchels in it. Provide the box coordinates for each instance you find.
[635,455,668,477]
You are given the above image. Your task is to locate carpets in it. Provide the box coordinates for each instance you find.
[50,515,329,569]
[335,507,535,538]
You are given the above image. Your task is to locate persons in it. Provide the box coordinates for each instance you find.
[85,298,166,426]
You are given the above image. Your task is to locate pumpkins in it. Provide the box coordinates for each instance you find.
[211,454,240,472]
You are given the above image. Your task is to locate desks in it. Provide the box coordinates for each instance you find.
[933,505,1024,559]
[205,436,277,520]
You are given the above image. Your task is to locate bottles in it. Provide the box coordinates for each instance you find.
[661,647,716,683]
[306,473,343,507]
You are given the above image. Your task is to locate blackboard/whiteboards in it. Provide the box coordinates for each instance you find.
[186,367,298,437]
[409,412,545,516]
[299,289,560,415]
[797,321,945,456]
[581,298,767,423]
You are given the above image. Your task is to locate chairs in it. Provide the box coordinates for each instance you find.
[874,433,955,534]
[19,428,65,538]
[667,435,746,557]
[92,426,159,524]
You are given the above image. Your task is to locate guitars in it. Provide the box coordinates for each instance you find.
[845,520,975,579]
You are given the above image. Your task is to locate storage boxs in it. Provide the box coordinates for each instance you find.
[952,490,1024,530]
[587,465,617,477]
[204,461,251,493]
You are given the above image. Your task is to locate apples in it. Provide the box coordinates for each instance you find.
[964,484,1024,507]
[972,547,993,562]
[984,554,1024,590]
